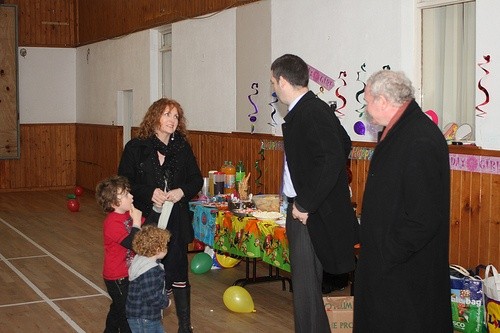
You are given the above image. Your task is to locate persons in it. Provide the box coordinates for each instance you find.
[119,98,204,333]
[271,54,360,333]
[95,177,156,333]
[125,225,172,333]
[352,71,453,333]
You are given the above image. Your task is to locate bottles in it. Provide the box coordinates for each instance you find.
[208,161,245,196]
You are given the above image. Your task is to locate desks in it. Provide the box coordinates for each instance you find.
[189,200,292,293]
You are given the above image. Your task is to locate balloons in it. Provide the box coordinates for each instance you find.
[223,286,256,313]
[190,252,213,274]
[73,187,84,197]
[68,199,80,212]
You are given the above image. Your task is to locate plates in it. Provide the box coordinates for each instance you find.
[253,212,283,220]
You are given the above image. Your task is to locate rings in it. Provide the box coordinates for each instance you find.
[300,219,302,221]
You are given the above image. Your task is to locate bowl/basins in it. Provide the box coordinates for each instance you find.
[253,194,280,211]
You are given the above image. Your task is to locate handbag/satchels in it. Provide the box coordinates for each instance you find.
[449,264,488,333]
[482,265,500,333]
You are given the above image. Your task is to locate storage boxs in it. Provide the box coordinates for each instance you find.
[253,195,280,212]
[323,296,353,333]
